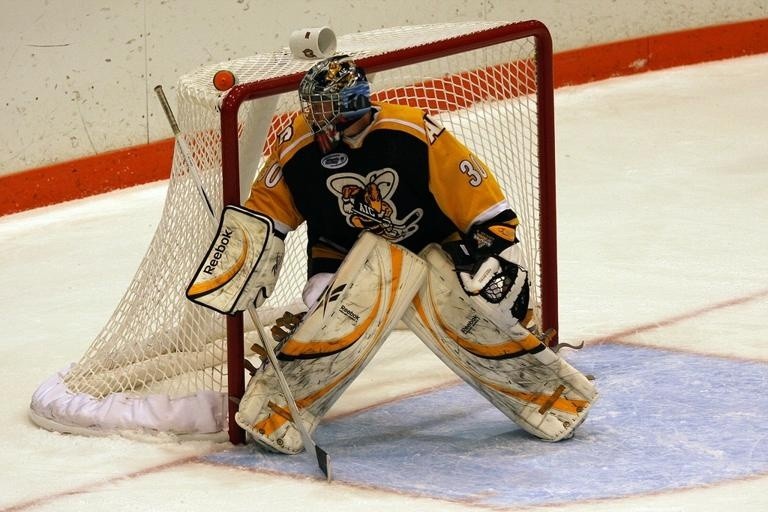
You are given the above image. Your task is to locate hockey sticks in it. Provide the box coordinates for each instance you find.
[156,83,334,481]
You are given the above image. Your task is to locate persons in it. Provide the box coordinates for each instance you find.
[184,53,601,454]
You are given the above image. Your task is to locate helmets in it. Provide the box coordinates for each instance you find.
[298,54,371,137]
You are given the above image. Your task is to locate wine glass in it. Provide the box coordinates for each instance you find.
[288,27,337,60]
